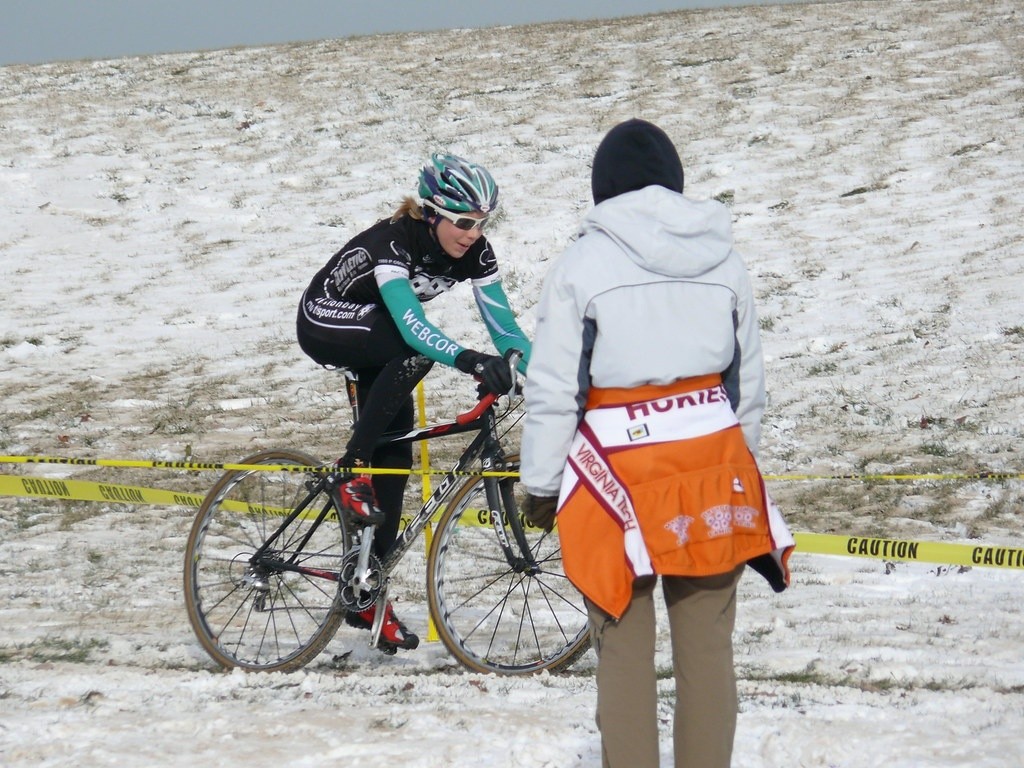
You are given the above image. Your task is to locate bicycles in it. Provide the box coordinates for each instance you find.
[183,347,593,677]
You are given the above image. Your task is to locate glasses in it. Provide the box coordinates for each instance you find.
[423,199,489,231]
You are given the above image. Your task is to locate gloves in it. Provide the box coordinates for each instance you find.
[455,349,513,393]
[521,493,557,533]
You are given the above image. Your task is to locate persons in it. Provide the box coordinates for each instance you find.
[520,118,798,768]
[296,152,533,651]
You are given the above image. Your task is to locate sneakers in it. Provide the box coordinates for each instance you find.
[331,458,385,524]
[345,600,420,650]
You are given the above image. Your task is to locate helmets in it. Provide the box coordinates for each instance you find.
[417,150,498,212]
